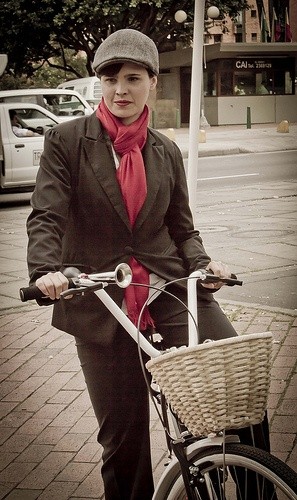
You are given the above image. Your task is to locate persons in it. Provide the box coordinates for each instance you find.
[25,27,277,500]
[9,109,40,136]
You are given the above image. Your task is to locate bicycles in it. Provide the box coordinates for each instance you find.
[20,263,297,500]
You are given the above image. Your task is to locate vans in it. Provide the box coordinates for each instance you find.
[0,88,95,125]
[56,77,103,110]
[0,102,67,189]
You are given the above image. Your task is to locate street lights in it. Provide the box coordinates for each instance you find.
[174,6,221,213]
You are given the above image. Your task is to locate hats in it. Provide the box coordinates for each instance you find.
[92,30,161,75]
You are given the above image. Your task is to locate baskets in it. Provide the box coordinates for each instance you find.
[144,330,274,436]
